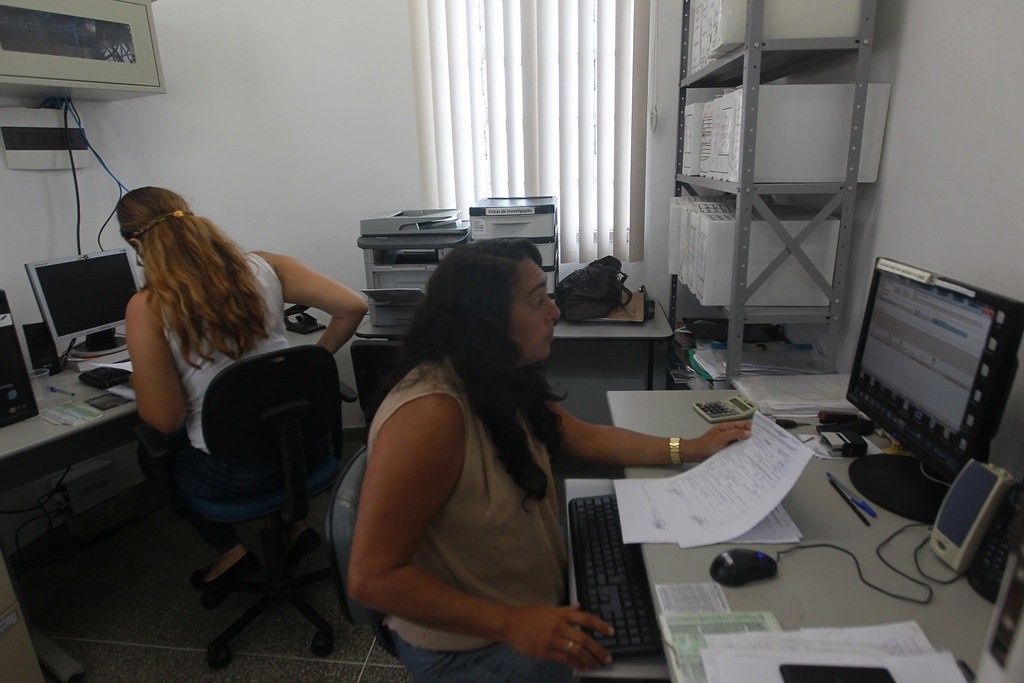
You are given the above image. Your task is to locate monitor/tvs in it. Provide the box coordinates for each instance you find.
[23,248,142,358]
[846,258,1024,523]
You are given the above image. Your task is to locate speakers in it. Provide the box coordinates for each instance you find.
[930,460,1014,575]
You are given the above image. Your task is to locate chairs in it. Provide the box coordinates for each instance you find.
[324,443,400,661]
[168,344,346,670]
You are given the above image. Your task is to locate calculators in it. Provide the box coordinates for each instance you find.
[691,395,755,423]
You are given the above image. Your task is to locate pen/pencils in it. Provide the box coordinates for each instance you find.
[50,386,74,397]
[827,470,876,527]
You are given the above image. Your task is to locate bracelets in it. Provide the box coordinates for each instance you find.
[669,437,683,466]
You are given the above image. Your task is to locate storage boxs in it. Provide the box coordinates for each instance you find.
[668,1,892,312]
[468,196,559,298]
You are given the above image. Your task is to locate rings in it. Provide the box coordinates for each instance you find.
[566,641,574,653]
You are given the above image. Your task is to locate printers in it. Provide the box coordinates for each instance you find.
[356,209,470,328]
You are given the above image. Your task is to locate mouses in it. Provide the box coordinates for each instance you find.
[709,549,777,588]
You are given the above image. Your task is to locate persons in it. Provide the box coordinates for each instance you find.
[118,186,367,592]
[339,235,754,683]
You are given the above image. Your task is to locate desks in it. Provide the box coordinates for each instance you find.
[353,297,676,474]
[566,390,1024,683]
[0,314,327,683]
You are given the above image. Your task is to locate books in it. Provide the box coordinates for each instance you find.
[732,373,858,418]
[690,341,829,391]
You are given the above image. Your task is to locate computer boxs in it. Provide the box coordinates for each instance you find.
[0,288,40,428]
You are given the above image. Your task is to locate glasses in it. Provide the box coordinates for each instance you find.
[136,252,143,269]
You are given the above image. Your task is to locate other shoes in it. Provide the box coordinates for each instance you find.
[286,529,321,563]
[190,552,253,588]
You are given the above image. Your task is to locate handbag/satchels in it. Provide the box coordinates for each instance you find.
[555,256,632,321]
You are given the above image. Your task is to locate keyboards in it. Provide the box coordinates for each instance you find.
[78,358,132,390]
[569,493,663,657]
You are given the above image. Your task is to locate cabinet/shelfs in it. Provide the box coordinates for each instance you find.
[664,0,879,390]
